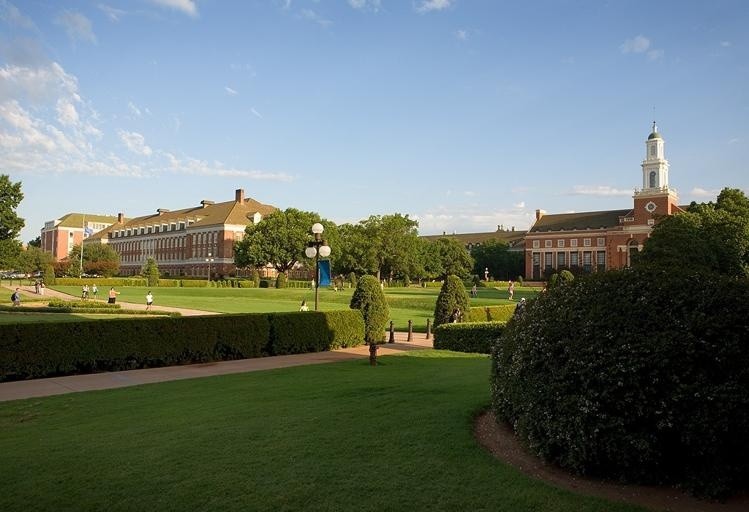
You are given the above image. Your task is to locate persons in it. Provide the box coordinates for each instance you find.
[472,284,477,298]
[146,291,153,310]
[509,283,515,300]
[108,287,120,303]
[12,288,21,307]
[299,300,310,312]
[82,284,98,301]
[35,278,45,295]
[311,278,315,290]
[449,309,460,323]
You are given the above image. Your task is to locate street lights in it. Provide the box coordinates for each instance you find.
[306,222,332,311]
[205,253,214,281]
